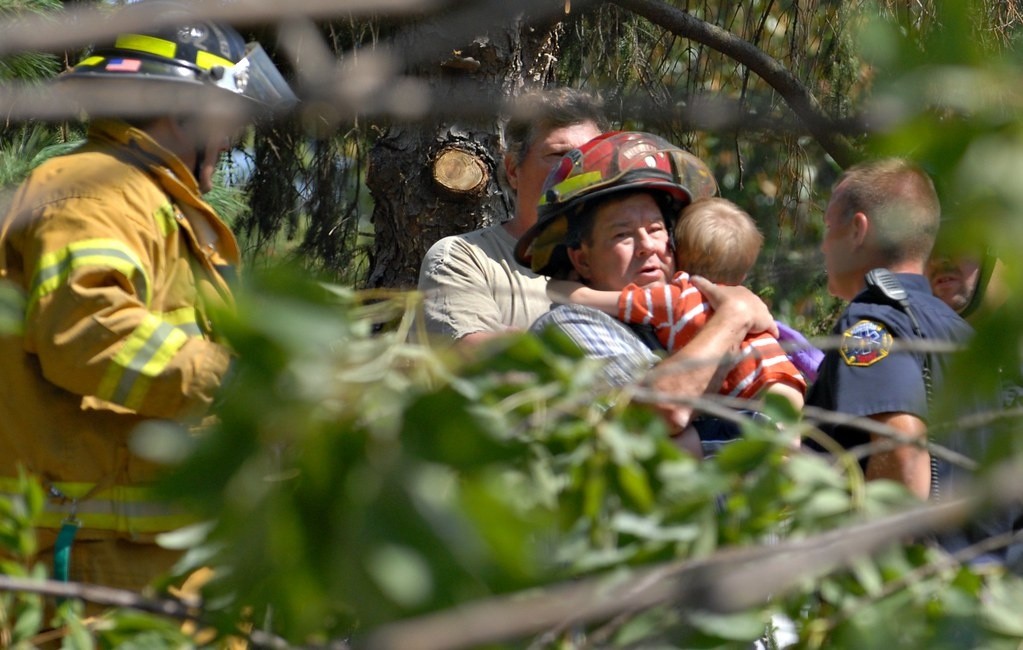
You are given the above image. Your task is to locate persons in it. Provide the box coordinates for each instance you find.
[514,132,781,436]
[416,87,606,377]
[0,0,250,650]
[798,157,1022,581]
[545,196,806,514]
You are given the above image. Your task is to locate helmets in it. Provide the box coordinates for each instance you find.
[51,14,275,115]
[513,128,694,277]
